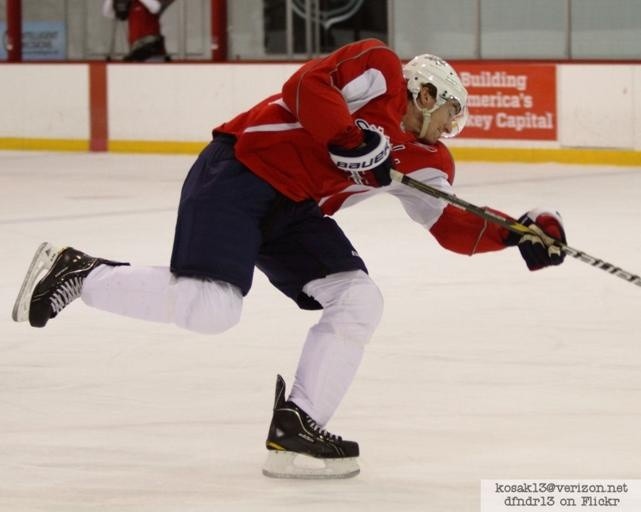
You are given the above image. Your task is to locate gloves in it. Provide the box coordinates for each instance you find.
[516,207,570,272]
[331,120,397,188]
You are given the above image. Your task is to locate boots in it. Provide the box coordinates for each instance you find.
[12,241,103,328]
[261,374,362,482]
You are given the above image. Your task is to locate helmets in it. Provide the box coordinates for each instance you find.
[402,53,469,138]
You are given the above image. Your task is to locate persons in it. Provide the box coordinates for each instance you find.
[25,36,571,460]
[100,0,177,59]
